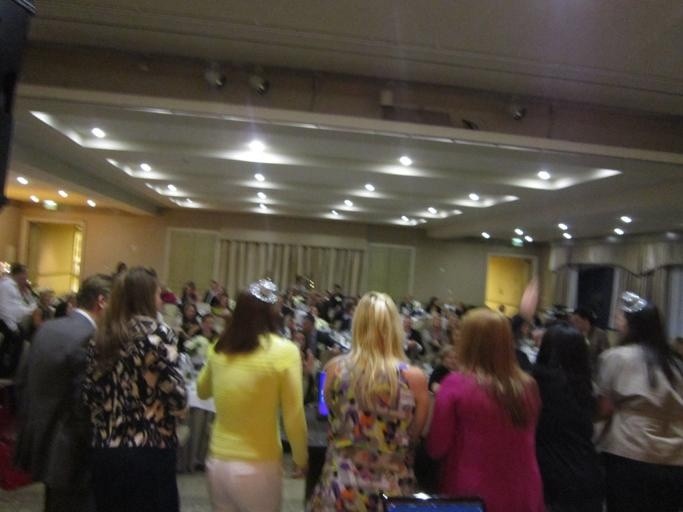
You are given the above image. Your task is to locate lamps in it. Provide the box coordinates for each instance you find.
[203,61,227,91]
[508,101,527,122]
[378,89,394,109]
[247,73,271,96]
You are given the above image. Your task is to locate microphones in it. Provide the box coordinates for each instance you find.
[25,282,40,299]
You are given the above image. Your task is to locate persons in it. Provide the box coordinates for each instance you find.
[55,290,78,318]
[594,289,683,512]
[33,288,58,340]
[515,273,603,512]
[425,306,548,511]
[302,290,428,512]
[390,294,467,399]
[17,273,118,512]
[503,306,622,368]
[157,278,231,476]
[274,284,358,407]
[74,263,186,511]
[195,280,310,511]
[0,260,37,379]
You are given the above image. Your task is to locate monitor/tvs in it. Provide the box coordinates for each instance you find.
[379,495,486,512]
[316,370,328,421]
